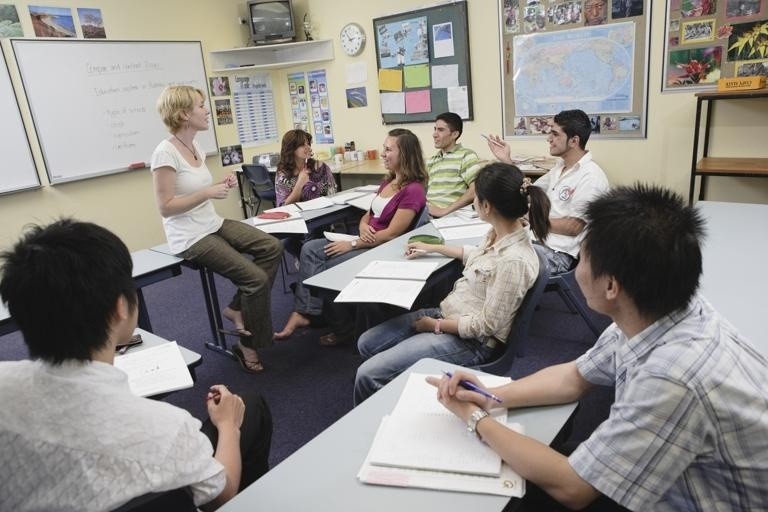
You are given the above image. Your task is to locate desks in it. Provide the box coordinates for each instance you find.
[216,357,578,512]
[150,184,387,361]
[695,200,768,371]
[0,249,184,337]
[302,202,494,356]
[341,159,555,186]
[0,328,202,401]
[233,159,369,220]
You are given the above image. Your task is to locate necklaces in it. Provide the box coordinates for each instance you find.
[173,133,198,161]
[295,162,304,173]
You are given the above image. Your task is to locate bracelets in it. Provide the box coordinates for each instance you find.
[434,318,445,335]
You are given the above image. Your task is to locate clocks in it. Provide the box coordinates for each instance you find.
[339,23,366,57]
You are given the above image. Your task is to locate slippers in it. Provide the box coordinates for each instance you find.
[319,332,338,346]
[232,344,265,374]
[218,328,255,337]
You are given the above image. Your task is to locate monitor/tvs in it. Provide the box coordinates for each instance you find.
[247,0,295,46]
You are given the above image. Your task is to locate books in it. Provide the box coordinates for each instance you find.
[111,339,195,398]
[429,208,494,240]
[357,372,527,498]
[333,260,440,311]
[253,184,381,234]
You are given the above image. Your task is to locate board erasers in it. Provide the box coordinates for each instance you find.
[130,163,145,170]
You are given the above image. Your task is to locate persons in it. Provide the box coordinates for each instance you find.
[274,129,338,272]
[584,0,608,27]
[487,109,610,278]
[426,180,768,512]
[0,217,272,511]
[271,128,429,340]
[414,112,481,229]
[150,85,282,374]
[503,0,581,33]
[352,162,550,409]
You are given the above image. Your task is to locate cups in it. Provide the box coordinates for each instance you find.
[366,150,377,160]
[350,151,357,161]
[344,152,350,161]
[357,152,364,161]
[332,154,344,164]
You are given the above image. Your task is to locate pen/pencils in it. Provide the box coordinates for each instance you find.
[410,249,426,253]
[206,385,228,400]
[482,134,504,149]
[305,156,308,171]
[442,369,502,404]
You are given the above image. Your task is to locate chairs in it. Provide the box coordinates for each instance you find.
[110,484,197,512]
[415,205,428,229]
[272,193,303,295]
[242,163,277,216]
[547,262,600,340]
[467,244,551,376]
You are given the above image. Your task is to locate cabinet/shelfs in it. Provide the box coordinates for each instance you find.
[689,89,768,210]
[209,39,334,73]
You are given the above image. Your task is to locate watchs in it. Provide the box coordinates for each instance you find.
[351,240,358,250]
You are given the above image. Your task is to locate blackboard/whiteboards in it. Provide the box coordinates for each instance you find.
[9,39,220,186]
[0,41,42,196]
